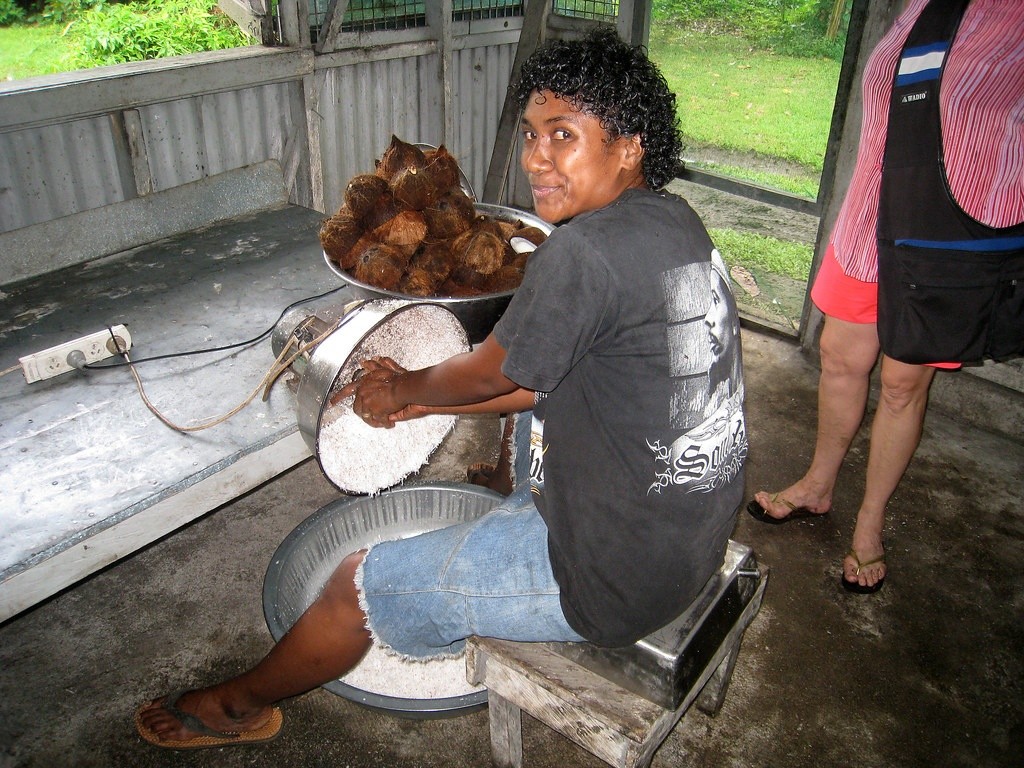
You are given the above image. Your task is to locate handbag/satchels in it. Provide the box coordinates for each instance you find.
[878,230,1024,364]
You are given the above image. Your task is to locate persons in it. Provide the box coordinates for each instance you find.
[133,22,751,749]
[753,0,1024,591]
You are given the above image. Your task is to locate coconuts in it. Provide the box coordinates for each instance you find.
[318,132,548,298]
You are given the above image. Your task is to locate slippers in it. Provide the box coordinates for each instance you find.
[747,492,829,525]
[842,540,887,594]
[467,463,497,483]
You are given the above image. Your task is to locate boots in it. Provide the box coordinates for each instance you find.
[134,689,284,750]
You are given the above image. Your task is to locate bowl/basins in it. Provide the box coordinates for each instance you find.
[323,202,558,346]
[262,480,509,720]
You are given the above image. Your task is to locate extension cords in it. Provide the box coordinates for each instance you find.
[19,324,130,384]
